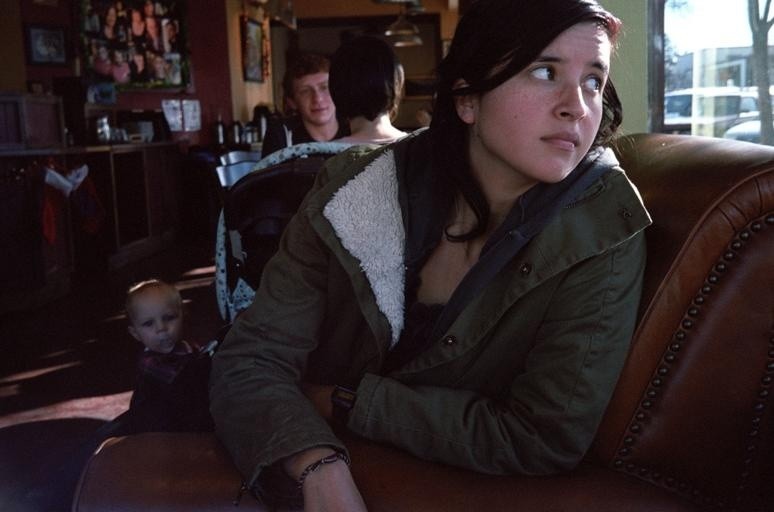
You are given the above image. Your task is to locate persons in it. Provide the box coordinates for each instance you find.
[207,0,654,511]
[326,33,409,147]
[122,276,222,437]
[261,55,340,162]
[89,0,184,89]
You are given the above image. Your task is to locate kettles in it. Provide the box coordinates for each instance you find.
[253,105,273,141]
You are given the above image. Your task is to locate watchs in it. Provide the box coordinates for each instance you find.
[327,361,365,429]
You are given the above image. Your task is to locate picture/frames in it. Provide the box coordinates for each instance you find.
[21,20,74,68]
[238,14,263,83]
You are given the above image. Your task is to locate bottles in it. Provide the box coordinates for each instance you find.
[213,119,242,145]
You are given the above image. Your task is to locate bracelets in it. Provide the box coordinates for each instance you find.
[294,451,352,494]
